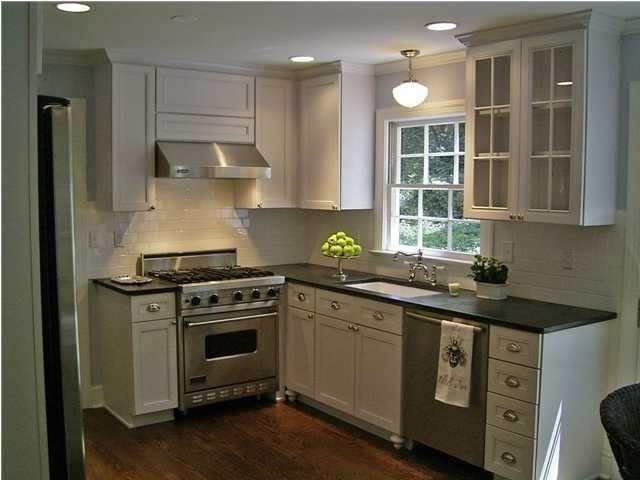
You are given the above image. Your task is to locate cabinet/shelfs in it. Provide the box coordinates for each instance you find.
[154,112,255,144]
[453,8,623,228]
[314,282,448,449]
[155,58,255,117]
[484,305,615,479]
[281,282,314,403]
[93,281,178,428]
[295,61,372,210]
[94,52,155,210]
[235,68,296,210]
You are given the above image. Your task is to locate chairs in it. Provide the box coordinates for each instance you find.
[599,382,640,480]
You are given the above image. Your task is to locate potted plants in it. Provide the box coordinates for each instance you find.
[467,255,509,300]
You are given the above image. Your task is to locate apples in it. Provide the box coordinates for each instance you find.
[321,232,362,256]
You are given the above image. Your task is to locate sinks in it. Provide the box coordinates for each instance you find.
[335,278,445,298]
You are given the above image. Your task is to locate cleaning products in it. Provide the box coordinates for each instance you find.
[448,279,460,297]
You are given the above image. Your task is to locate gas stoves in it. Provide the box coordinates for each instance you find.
[146,265,286,309]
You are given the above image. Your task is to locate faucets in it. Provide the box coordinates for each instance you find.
[392,249,424,265]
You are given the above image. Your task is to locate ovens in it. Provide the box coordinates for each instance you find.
[174,299,280,411]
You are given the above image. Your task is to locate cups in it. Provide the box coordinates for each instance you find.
[448,282,462,296]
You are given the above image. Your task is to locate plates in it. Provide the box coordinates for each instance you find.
[111,273,152,285]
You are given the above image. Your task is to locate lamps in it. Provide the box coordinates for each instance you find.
[392,50,429,108]
